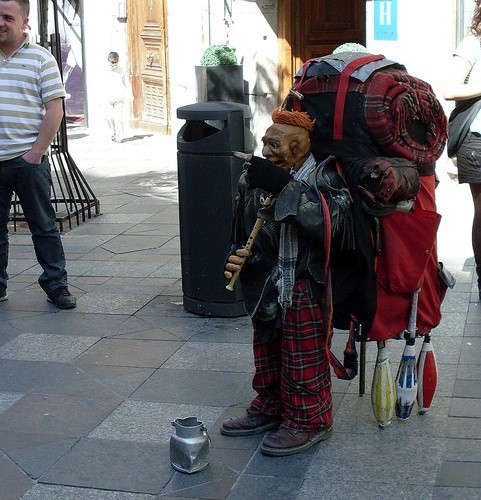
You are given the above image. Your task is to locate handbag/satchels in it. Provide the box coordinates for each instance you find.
[367,208,449,340]
[448,96,481,157]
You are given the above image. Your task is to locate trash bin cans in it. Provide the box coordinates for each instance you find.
[177,101,257,318]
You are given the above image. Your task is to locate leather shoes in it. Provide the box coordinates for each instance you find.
[260,426,332,456]
[220,407,279,435]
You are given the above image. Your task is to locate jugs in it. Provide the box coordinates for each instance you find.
[170,416,212,474]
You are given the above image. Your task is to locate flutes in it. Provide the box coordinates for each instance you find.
[224,194,272,293]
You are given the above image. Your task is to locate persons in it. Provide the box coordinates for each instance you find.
[0,0,77,310]
[442,0,481,300]
[220,107,354,455]
[97,52,134,143]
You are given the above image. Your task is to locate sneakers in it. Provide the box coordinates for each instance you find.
[47,285,76,309]
[0,288,8,301]
